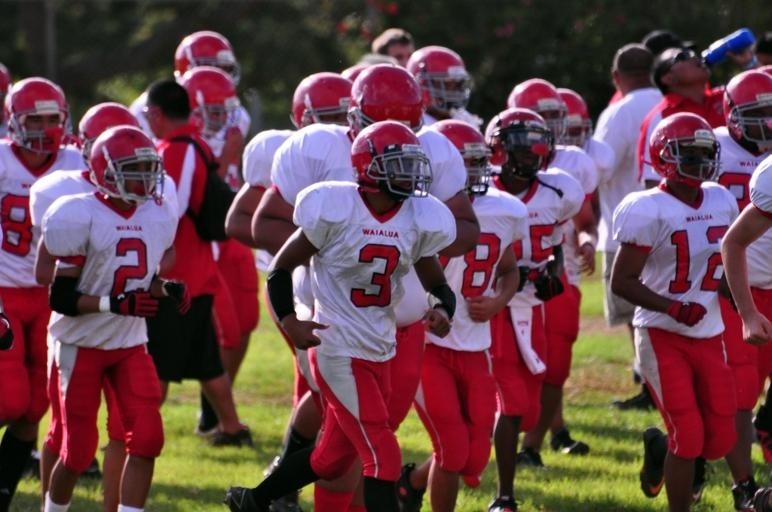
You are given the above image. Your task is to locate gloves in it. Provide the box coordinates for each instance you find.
[664,298,709,329]
[515,263,531,292]
[0,310,16,352]
[164,277,195,317]
[533,274,565,303]
[108,285,163,320]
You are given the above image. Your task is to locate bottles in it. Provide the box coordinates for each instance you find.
[700,25,754,69]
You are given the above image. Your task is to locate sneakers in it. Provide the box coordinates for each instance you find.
[261,454,305,512]
[487,494,518,512]
[727,475,762,512]
[691,453,707,504]
[637,426,669,498]
[210,425,257,447]
[753,482,772,512]
[394,461,427,512]
[220,484,273,512]
[517,443,552,472]
[547,428,592,456]
[192,409,223,437]
[611,383,659,411]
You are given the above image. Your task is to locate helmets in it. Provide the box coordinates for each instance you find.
[555,86,591,125]
[289,71,354,130]
[174,29,238,78]
[429,118,494,160]
[406,44,473,107]
[0,61,13,113]
[351,63,423,131]
[647,109,719,180]
[338,63,372,86]
[347,119,421,194]
[87,124,161,189]
[721,63,772,143]
[485,106,551,167]
[178,65,240,117]
[3,76,72,132]
[506,77,569,114]
[76,99,144,148]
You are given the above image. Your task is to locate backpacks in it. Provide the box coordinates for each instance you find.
[167,132,240,244]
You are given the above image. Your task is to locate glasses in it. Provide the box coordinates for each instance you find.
[666,50,696,71]
